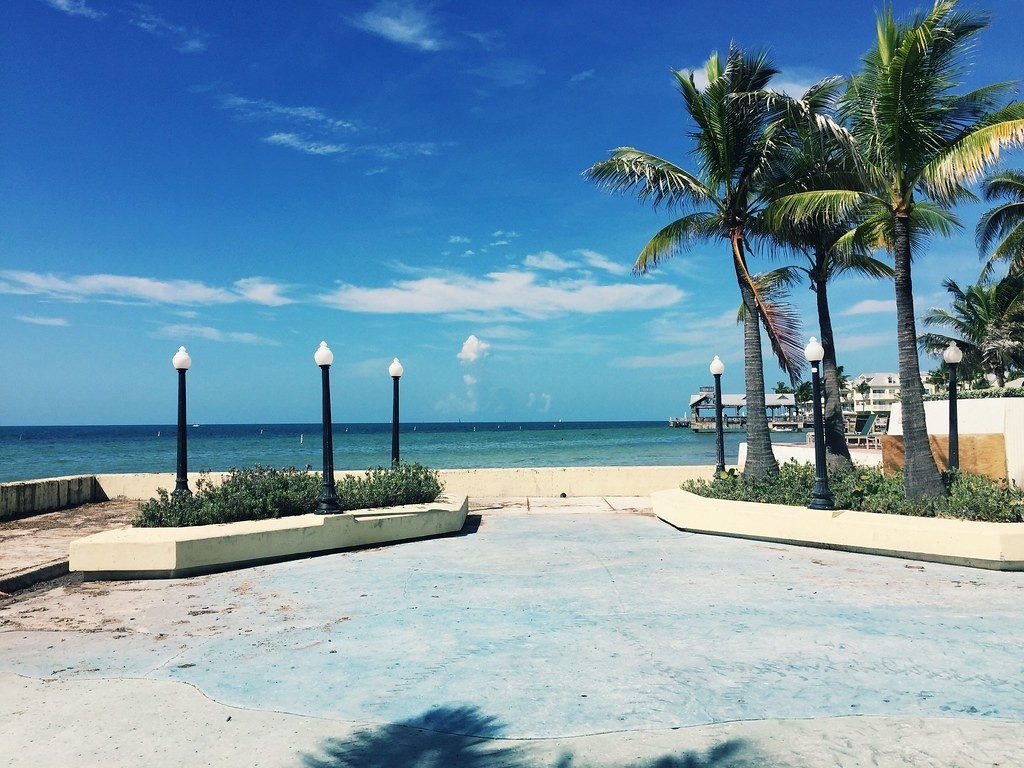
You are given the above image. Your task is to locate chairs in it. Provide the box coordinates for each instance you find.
[806,414,890,450]
